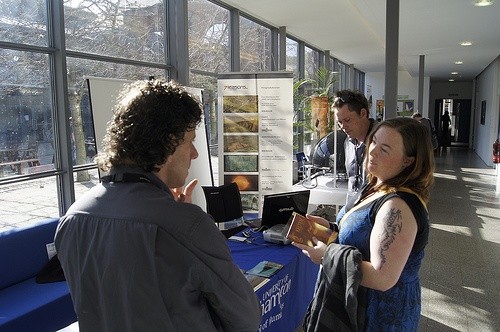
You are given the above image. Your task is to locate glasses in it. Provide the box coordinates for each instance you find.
[334,96,362,103]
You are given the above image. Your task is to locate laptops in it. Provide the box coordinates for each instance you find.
[249,191,310,233]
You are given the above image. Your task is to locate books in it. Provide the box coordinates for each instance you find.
[281,211,339,248]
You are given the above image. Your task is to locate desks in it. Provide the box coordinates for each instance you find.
[226,229,320,332]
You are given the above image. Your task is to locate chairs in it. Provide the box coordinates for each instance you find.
[201,182,248,238]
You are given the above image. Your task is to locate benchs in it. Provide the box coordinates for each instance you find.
[0,216,80,332]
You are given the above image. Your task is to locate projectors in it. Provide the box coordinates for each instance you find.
[262,224,292,245]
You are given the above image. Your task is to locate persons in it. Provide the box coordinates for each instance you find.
[54,79,262,332]
[412,111,452,149]
[291,116,435,332]
[331,89,380,200]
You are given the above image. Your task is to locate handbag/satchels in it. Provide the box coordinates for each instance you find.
[432,132,438,150]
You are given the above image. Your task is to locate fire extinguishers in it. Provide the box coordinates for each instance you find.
[492,139,500,163]
